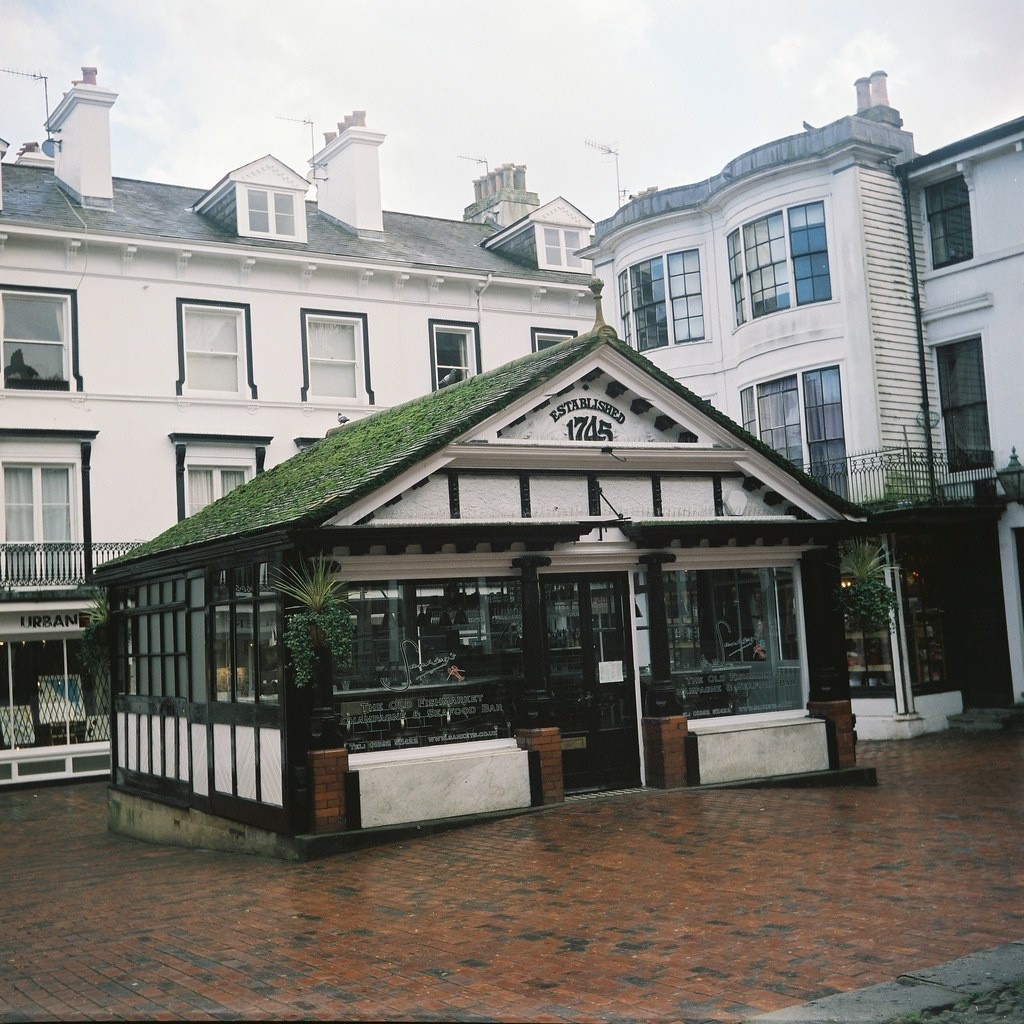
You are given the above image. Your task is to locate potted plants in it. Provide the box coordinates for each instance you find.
[271,552,358,690]
[78,587,108,666]
[828,535,904,635]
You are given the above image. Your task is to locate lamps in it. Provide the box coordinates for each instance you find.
[382,609,469,633]
